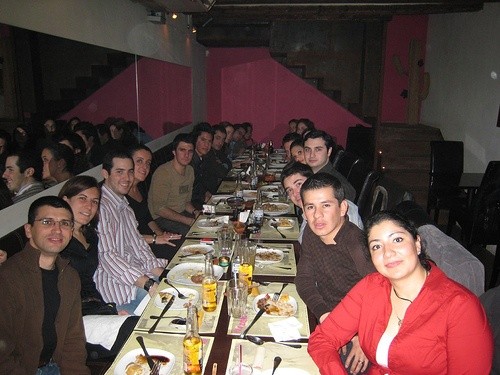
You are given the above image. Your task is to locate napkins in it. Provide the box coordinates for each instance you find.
[217,210,251,222]
[265,317,303,343]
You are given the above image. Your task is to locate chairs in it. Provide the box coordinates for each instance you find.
[327,141,500,375]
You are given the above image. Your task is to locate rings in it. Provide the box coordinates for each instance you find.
[359,361,364,364]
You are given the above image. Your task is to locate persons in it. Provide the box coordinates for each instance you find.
[282,117,378,375]
[0,120,253,375]
[307,211,494,375]
[0,116,153,209]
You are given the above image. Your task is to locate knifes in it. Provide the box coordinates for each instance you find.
[240,304,269,339]
[148,295,175,334]
[136,336,160,375]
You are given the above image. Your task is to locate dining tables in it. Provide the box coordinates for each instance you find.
[97,137,316,375]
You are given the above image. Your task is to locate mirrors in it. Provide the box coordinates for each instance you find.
[0,24,194,216]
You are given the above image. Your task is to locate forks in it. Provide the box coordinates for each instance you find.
[273,282,289,303]
[150,360,161,375]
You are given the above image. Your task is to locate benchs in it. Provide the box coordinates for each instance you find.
[0,142,174,259]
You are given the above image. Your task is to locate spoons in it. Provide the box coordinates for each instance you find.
[245,335,302,348]
[164,278,187,299]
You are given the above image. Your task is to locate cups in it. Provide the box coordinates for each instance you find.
[216,227,260,318]
[229,362,253,375]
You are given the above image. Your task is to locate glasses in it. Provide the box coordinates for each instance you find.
[36,218,74,230]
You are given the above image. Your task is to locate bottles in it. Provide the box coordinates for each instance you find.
[235,141,274,227]
[239,238,252,295]
[183,306,203,375]
[201,252,218,312]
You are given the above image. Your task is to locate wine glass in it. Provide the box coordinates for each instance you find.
[227,196,243,221]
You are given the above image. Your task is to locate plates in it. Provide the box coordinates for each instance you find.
[242,189,257,199]
[268,367,309,374]
[255,248,284,263]
[268,217,295,229]
[166,262,225,286]
[154,287,200,309]
[259,149,292,215]
[114,347,175,375]
[181,244,215,259]
[253,293,297,317]
[197,154,249,228]
